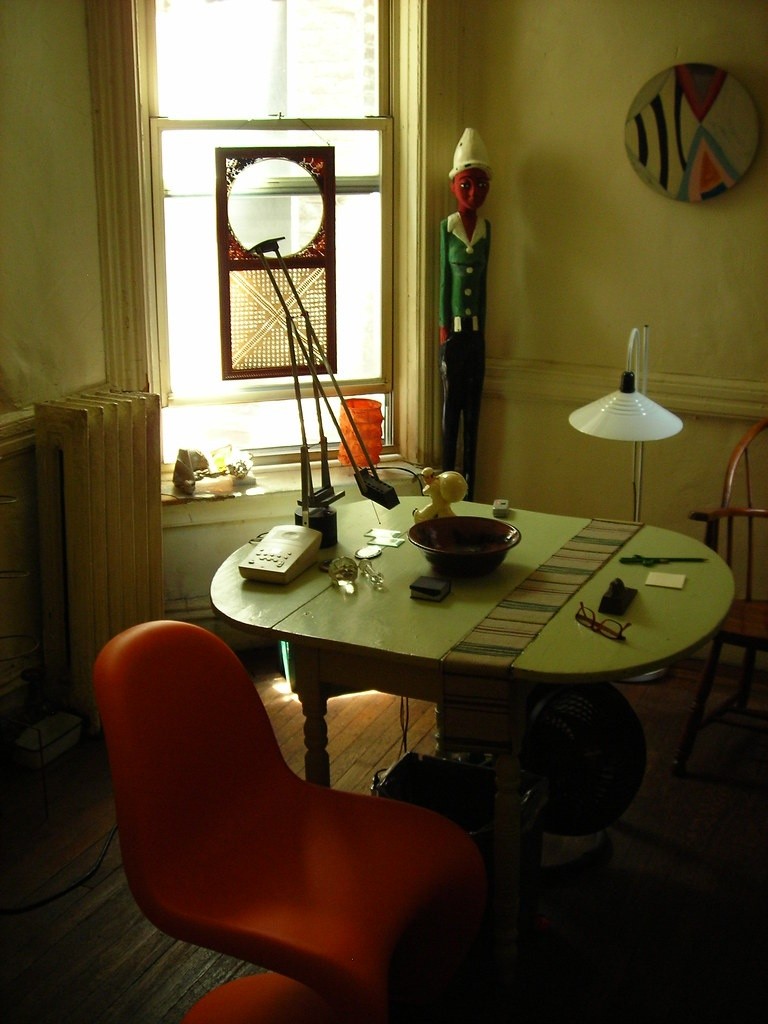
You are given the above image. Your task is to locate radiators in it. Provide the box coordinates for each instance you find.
[32,388,166,746]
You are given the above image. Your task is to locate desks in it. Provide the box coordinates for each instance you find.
[209,496,736,991]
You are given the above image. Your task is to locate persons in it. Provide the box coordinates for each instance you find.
[438,127,493,499]
[413,467,455,523]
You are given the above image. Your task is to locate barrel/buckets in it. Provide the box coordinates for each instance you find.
[338,398,383,467]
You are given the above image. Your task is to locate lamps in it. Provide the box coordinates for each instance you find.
[567,327,684,683]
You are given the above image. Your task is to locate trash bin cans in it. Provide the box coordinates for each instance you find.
[378,748,546,932]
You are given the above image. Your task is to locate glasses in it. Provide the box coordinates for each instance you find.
[575,601,632,641]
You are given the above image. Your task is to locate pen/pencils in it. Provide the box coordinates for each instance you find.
[620,555,712,564]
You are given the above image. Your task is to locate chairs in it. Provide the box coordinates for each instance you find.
[95,620,488,1024]
[672,419,767,773]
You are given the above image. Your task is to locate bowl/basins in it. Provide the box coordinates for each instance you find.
[408,516,521,576]
